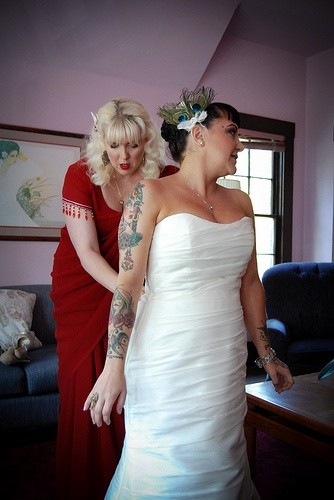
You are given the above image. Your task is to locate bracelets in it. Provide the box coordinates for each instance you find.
[256,351,275,368]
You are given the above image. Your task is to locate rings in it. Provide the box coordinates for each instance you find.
[91,393,98,409]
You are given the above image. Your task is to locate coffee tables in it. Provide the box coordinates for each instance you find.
[245,371,334,479]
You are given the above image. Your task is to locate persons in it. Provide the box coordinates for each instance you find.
[50,97,180,500]
[83,86,296,500]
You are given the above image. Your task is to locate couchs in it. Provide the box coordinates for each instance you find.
[0,285,59,432]
[263,260,334,380]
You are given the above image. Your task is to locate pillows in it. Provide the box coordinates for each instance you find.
[0,288,43,351]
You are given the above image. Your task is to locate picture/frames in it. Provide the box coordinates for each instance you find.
[0,123,89,241]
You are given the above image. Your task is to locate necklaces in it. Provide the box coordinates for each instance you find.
[179,171,219,211]
[111,167,135,205]
